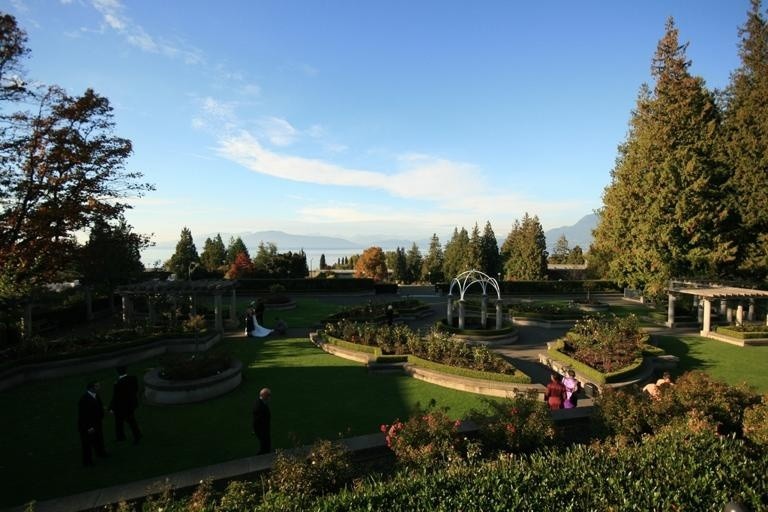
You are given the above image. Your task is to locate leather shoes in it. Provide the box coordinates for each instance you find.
[132,436,142,446]
[97,453,111,459]
[85,461,96,468]
[113,438,126,444]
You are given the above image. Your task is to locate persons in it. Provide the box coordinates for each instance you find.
[106,361,141,445]
[79,381,104,460]
[275,317,288,334]
[562,370,580,408]
[251,388,272,448]
[544,372,567,409]
[245,297,274,338]
